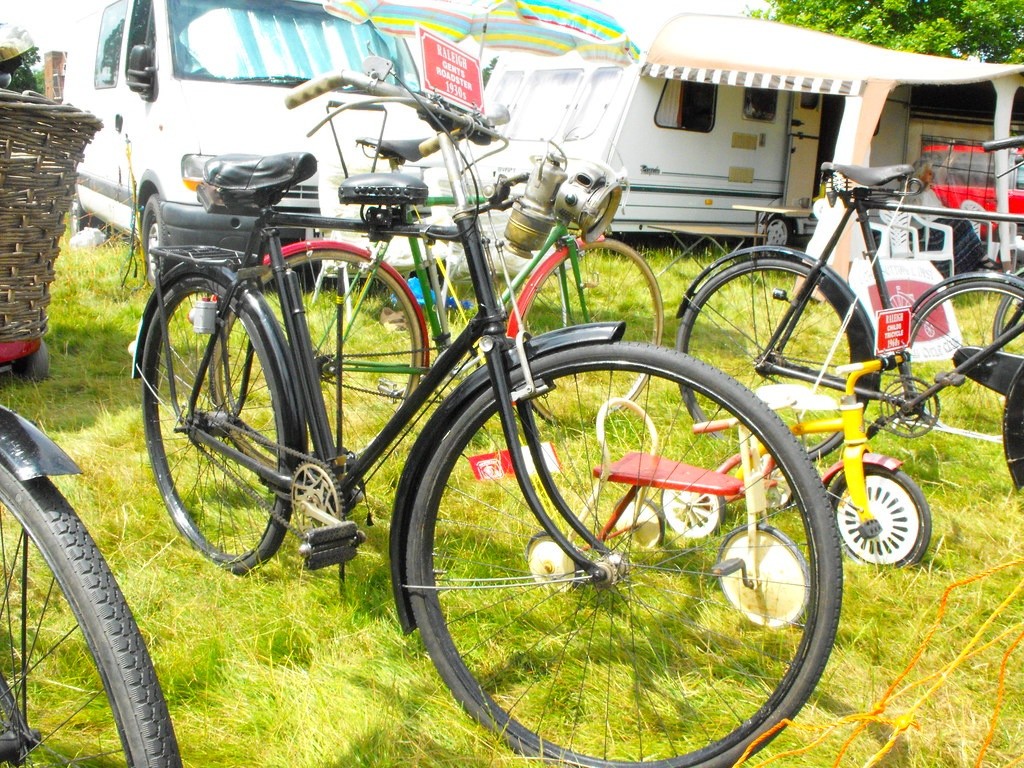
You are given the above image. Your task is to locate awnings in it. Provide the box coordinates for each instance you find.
[652,12,1024,282]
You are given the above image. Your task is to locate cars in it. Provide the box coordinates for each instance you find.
[917,142,1024,268]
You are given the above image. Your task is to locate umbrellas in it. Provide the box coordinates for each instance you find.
[316,0,641,66]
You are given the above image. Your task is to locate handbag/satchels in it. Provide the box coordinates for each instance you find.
[796,196,850,299]
[847,232,960,361]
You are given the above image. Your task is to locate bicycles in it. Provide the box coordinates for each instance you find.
[122,71,846,768]
[664,136,1024,442]
[3,409,183,767]
[205,105,667,457]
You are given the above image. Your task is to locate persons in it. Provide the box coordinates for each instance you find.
[900,158,1001,274]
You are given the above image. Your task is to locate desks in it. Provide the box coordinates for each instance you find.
[732,204,813,259]
[644,225,768,299]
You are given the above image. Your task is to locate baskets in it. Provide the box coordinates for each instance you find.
[0,83,105,343]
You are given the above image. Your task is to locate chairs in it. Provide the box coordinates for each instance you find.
[961,200,1017,283]
[813,198,890,259]
[879,200,954,287]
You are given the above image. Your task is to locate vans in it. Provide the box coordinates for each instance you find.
[63,1,467,296]
[481,50,829,263]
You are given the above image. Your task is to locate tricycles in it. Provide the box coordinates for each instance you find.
[660,350,938,572]
[523,396,809,624]
